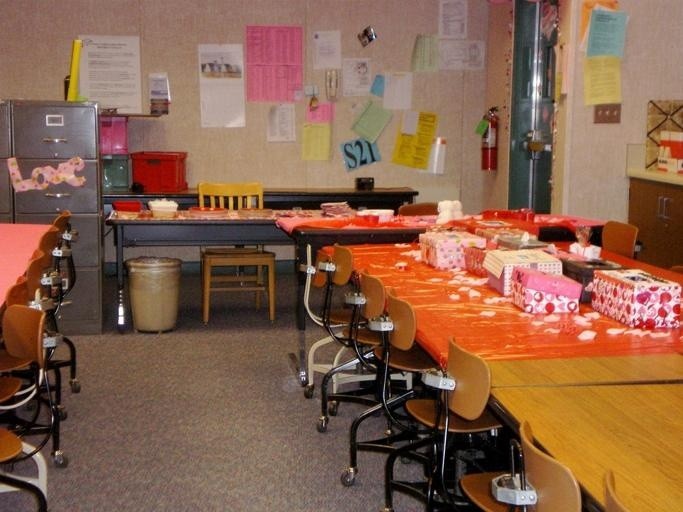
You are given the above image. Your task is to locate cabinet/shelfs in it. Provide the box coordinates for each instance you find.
[0,99,104,336]
[629,177,683,275]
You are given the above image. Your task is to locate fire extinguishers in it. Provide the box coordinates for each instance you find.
[480,105,500,170]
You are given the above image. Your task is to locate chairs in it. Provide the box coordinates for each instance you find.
[294,243,366,397]
[460,417,582,510]
[381,336,502,512]
[195,180,276,324]
[603,222,639,259]
[0,209,79,512]
[311,244,381,416]
[314,272,387,434]
[340,290,437,488]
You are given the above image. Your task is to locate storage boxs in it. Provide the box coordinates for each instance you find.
[656,131,683,174]
[97,113,190,193]
[417,222,682,328]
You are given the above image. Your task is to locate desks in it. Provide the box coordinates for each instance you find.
[98,187,420,277]
[104,210,444,334]
[322,244,683,389]
[490,382,682,512]
